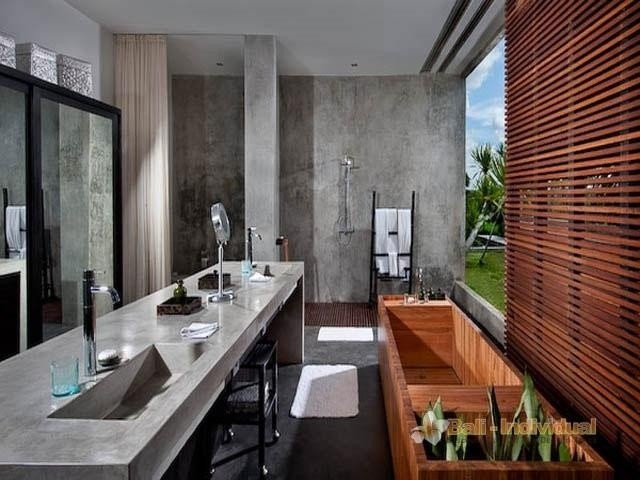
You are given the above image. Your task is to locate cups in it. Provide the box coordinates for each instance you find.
[241,260,251,273]
[50,357,79,397]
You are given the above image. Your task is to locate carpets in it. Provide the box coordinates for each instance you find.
[289,301,374,419]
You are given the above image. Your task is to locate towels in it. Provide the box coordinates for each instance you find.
[375,207,411,281]
[5,203,25,259]
[179,322,217,339]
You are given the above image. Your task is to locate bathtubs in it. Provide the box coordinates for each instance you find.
[375,290,532,391]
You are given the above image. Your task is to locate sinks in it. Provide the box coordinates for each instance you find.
[45,341,213,421]
[239,262,292,285]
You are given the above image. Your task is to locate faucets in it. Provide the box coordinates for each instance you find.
[414,267,425,298]
[246,227,262,269]
[82,269,120,377]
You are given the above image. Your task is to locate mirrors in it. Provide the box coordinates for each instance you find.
[1,65,123,362]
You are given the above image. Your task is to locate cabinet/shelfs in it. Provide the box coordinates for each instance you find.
[203,340,281,476]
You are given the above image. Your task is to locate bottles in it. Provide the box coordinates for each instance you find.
[174,279,187,304]
